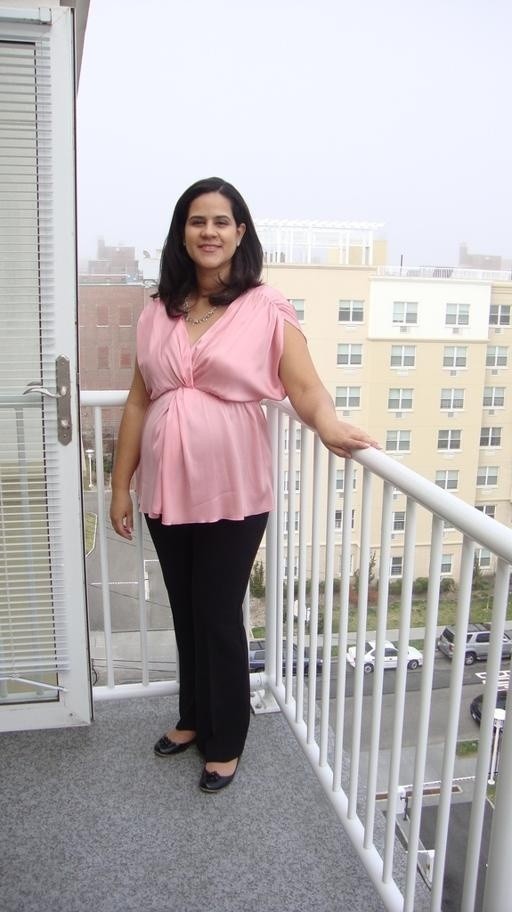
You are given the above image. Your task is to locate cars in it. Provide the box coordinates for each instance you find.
[469,689,507,733]
[346,639,423,674]
[250,649,322,677]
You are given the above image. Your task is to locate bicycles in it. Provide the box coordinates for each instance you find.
[92,659,99,686]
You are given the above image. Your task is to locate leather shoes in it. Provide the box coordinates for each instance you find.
[154,735,201,757]
[198,754,241,795]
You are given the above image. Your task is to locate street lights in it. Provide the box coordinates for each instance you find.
[487,708,504,787]
[85,448,95,489]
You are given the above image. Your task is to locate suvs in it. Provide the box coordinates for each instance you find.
[436,623,511,666]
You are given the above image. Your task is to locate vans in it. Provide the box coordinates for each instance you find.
[248,638,305,658]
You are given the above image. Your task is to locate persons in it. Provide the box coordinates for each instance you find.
[108,177,381,793]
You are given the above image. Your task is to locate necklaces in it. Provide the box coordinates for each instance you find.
[180,294,227,324]
